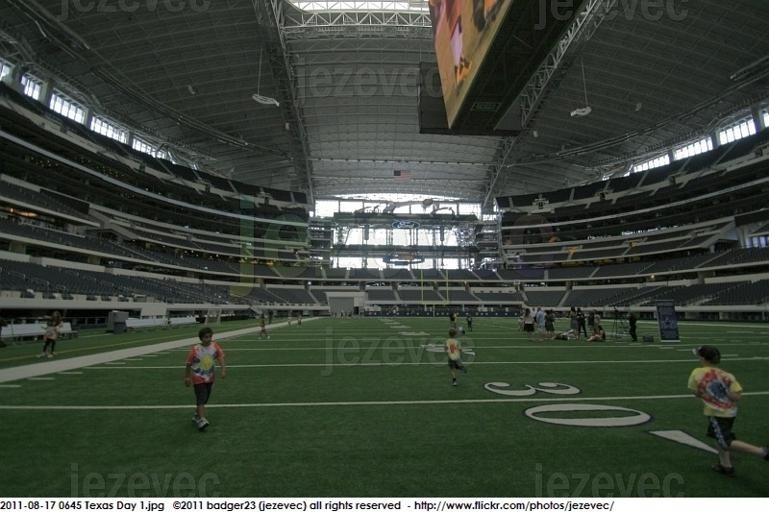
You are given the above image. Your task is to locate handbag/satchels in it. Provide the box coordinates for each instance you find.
[186,377,191,381]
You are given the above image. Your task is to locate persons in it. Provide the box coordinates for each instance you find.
[257,309,303,340]
[34,310,64,358]
[427,0,506,94]
[449,309,459,330]
[328,308,354,320]
[463,312,472,332]
[443,327,468,386]
[185,326,228,431]
[686,343,769,477]
[518,304,639,342]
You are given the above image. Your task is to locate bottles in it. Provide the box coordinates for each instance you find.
[692,345,720,364]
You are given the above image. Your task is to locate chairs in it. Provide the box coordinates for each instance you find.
[451,378,457,387]
[191,412,210,430]
[0,78,768,307]
[713,464,734,477]
